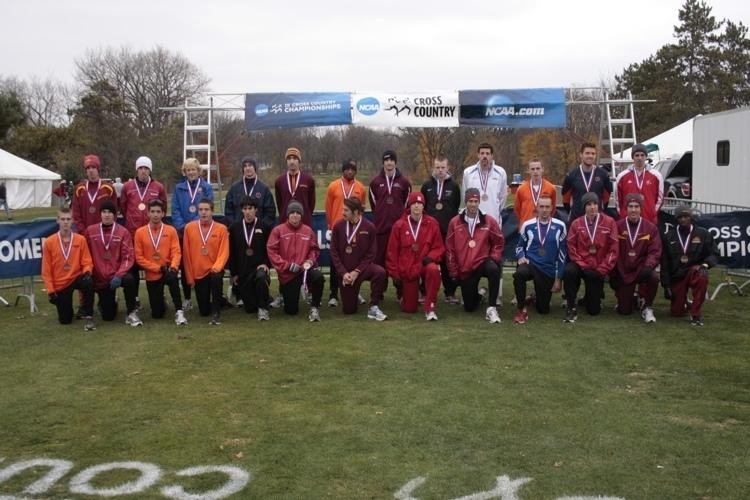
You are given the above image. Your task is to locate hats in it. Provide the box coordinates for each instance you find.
[465,188,480,202]
[675,207,693,221]
[408,192,426,208]
[625,193,642,206]
[286,202,304,219]
[100,201,116,216]
[83,154,101,172]
[383,150,397,163]
[135,156,153,173]
[581,193,600,208]
[342,158,358,171]
[632,144,647,158]
[285,147,302,164]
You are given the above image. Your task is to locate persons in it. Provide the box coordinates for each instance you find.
[382,95,402,118]
[82,200,145,327]
[513,143,664,325]
[170,155,215,313]
[0,180,14,221]
[659,204,721,329]
[39,206,97,334]
[324,144,507,324]
[119,155,169,314]
[394,97,413,117]
[70,154,118,320]
[225,147,325,322]
[133,197,189,327]
[183,196,231,329]
[52,176,134,206]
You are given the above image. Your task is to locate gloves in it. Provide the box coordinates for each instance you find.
[638,268,652,283]
[609,272,618,290]
[289,262,301,273]
[162,269,178,284]
[695,263,709,275]
[81,273,93,282]
[582,269,601,279]
[110,276,122,290]
[421,256,432,265]
[48,292,59,304]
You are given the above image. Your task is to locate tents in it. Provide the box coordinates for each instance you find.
[0,147,61,210]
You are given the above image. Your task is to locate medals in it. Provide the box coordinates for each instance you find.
[138,203,146,211]
[200,245,210,258]
[101,250,112,261]
[680,255,688,264]
[88,205,97,213]
[63,263,71,272]
[151,250,162,261]
[188,205,197,214]
